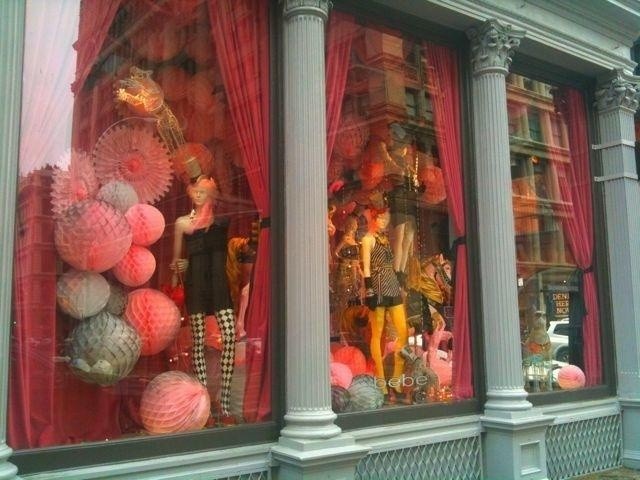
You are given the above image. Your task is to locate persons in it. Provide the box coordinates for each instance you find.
[361,202,426,393]
[334,213,366,333]
[169,174,249,427]
[380,125,418,277]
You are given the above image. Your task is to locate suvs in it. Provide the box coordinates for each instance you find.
[546,318,570,363]
